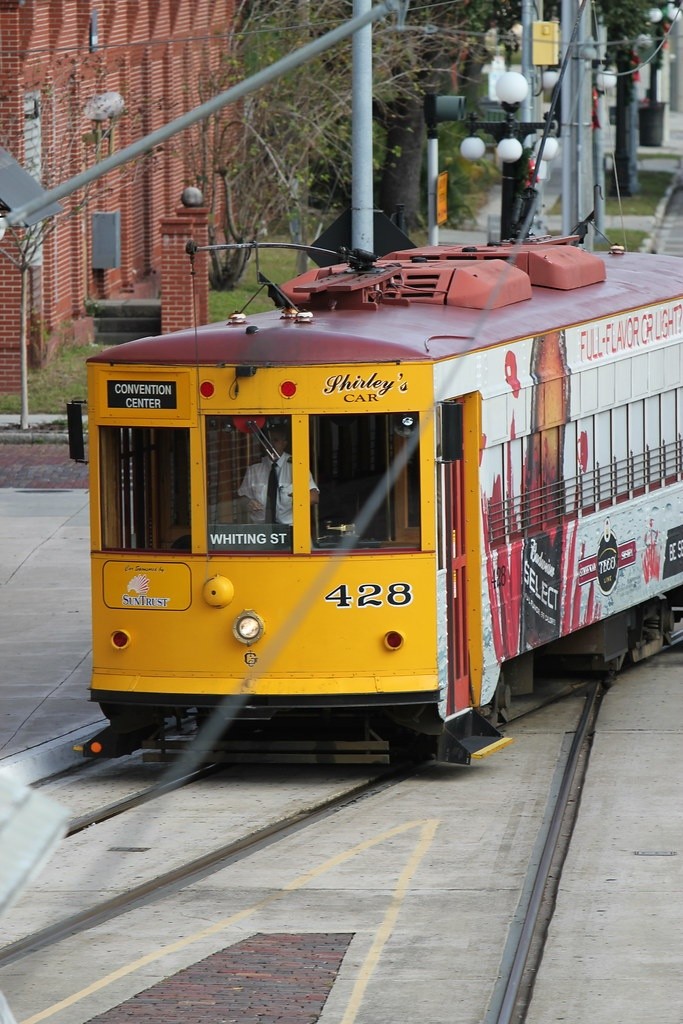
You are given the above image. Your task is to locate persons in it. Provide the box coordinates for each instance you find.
[237,427,323,551]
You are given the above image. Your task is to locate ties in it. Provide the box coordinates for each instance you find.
[265,463,279,524]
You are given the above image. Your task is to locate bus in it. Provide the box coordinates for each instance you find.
[64,233,683,769]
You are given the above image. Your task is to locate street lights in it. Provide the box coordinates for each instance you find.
[459,71,560,248]
[542,1,682,241]
